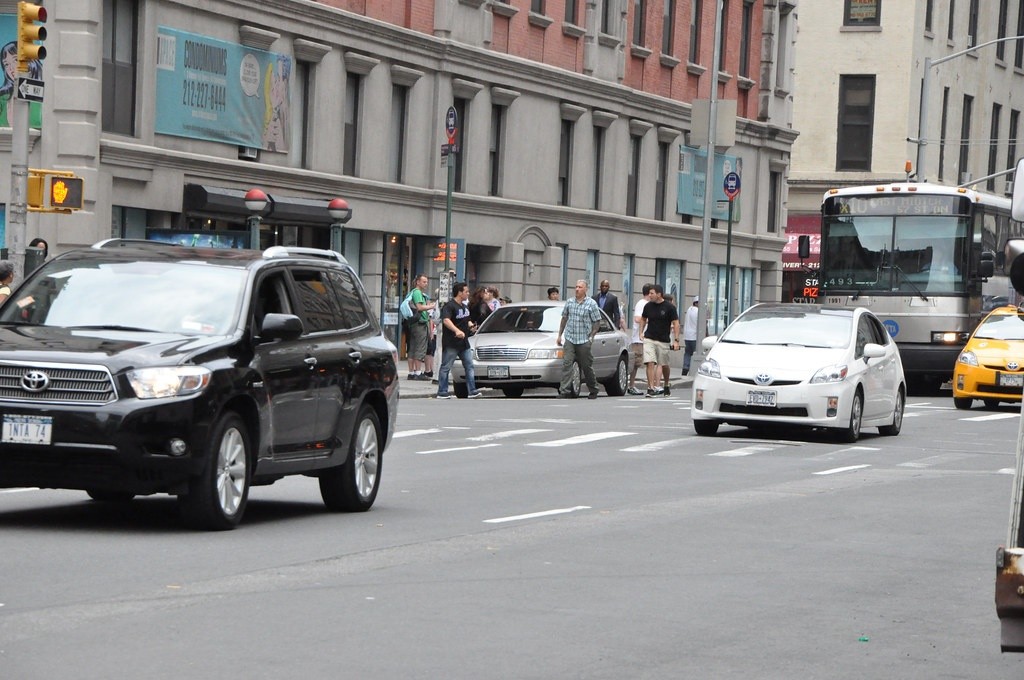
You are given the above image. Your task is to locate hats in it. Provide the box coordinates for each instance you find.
[692,296,707,306]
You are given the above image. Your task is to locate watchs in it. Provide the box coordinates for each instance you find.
[675,339,680,342]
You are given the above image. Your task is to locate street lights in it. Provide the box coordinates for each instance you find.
[246,189,270,250]
[327,199,353,256]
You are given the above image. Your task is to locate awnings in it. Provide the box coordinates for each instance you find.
[781,232,822,271]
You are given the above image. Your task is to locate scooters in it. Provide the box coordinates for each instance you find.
[48,176,85,211]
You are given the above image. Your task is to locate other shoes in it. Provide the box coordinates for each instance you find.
[555,392,574,399]
[681,370,690,376]
[437,392,451,400]
[588,391,599,400]
[407,374,414,379]
[467,391,482,398]
[413,372,431,381]
[423,370,433,378]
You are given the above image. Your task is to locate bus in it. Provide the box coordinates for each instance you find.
[816,183,1024,396]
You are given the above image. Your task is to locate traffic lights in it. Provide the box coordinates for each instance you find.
[15,1,50,76]
[14,78,45,103]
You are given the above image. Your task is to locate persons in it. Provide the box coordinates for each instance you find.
[0,259,15,305]
[467,285,512,338]
[627,283,653,395]
[403,271,440,381]
[437,282,483,399]
[556,279,602,399]
[639,285,681,398]
[589,279,620,330]
[547,287,559,300]
[682,296,699,376]
[29,238,48,258]
[919,247,959,276]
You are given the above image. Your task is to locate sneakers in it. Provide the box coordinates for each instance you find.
[654,386,665,395]
[663,386,672,397]
[646,387,655,397]
[627,387,644,395]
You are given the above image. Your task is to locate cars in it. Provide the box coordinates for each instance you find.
[451,297,634,400]
[948,306,1024,409]
[690,305,907,444]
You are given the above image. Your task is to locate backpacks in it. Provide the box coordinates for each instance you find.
[398,288,423,326]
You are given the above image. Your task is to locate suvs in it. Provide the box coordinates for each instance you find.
[0,238,400,531]
